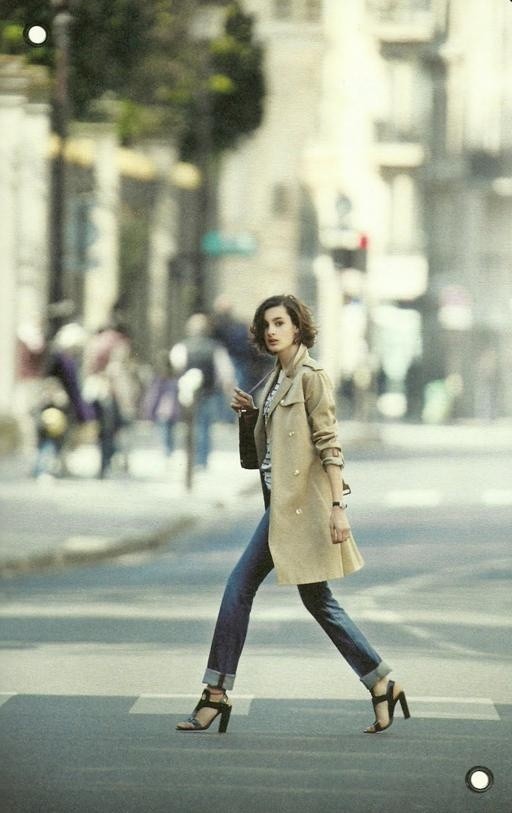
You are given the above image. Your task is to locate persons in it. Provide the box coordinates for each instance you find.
[177,294,410,733]
[14,293,266,480]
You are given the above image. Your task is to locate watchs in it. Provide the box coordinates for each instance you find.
[334,502,348,509]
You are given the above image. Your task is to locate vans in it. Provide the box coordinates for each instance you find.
[175,690,232,733]
[364,679,410,733]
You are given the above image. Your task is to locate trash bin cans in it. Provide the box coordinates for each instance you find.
[334,502,348,509]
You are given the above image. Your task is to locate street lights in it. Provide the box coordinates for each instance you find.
[238,407,260,470]
